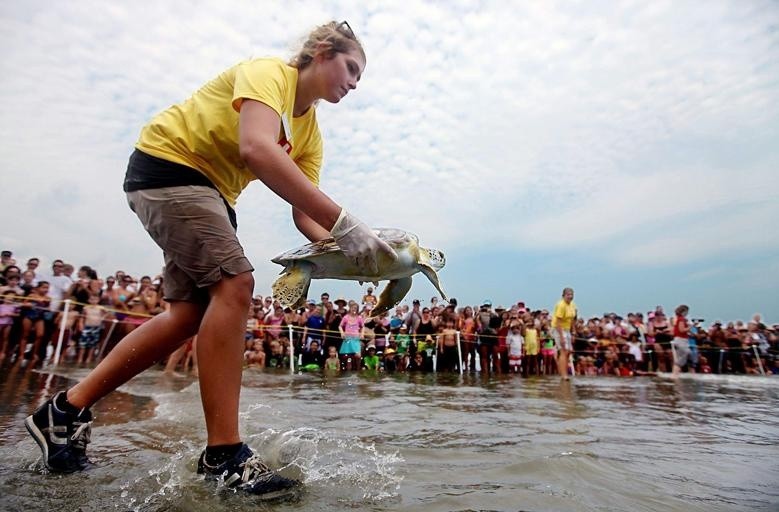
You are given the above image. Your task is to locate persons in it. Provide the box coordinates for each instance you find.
[24,21,398,503]
[0,249,778,383]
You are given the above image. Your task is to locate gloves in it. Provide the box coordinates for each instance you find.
[329,207,398,276]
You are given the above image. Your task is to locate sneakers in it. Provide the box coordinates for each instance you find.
[197,444,298,501]
[25,391,98,474]
